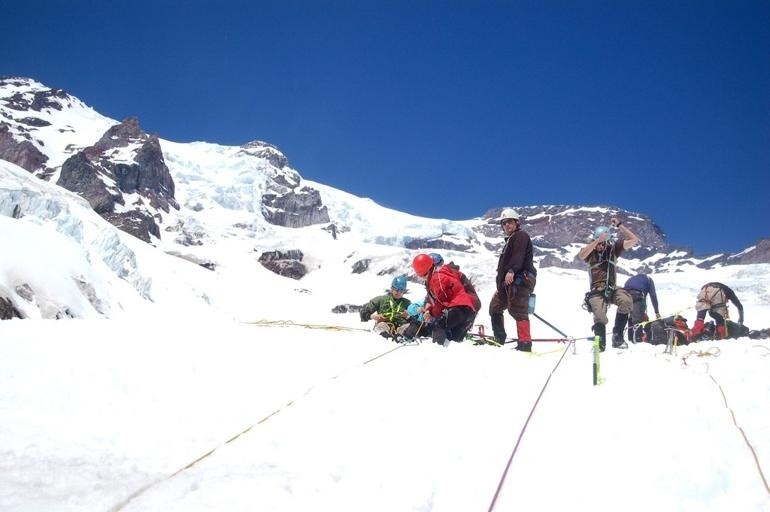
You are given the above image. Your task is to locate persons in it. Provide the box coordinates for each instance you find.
[624,274,661,341]
[489,208,537,353]
[580,218,634,353]
[413,253,482,342]
[360,276,412,343]
[692,282,744,340]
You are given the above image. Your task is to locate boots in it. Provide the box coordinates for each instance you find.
[690,319,705,340]
[590,323,608,352]
[716,324,726,340]
[612,312,629,349]
[514,319,532,352]
[491,314,507,346]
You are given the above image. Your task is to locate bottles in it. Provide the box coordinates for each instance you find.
[527,294,536,315]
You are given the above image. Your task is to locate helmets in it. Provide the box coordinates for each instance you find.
[392,277,407,291]
[412,252,443,276]
[592,226,611,242]
[500,208,519,223]
